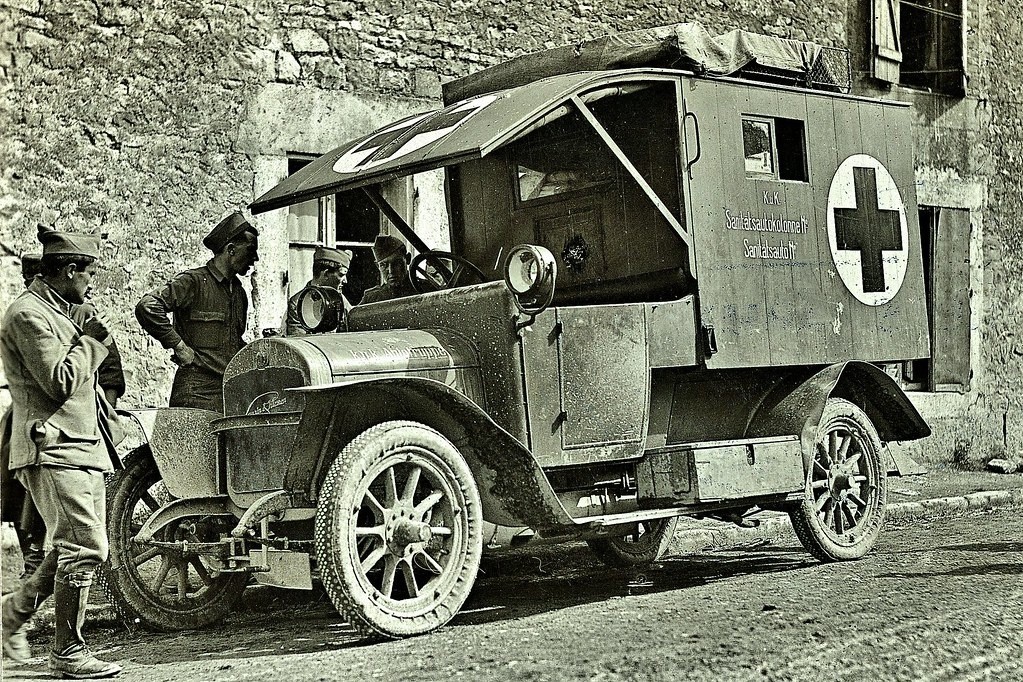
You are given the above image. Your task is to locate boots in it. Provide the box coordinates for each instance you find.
[47,568,121,676]
[3,560,57,662]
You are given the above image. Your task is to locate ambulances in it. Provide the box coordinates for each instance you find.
[122,45,935,645]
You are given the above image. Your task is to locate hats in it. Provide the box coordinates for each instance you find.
[372,234,405,262]
[204,213,249,253]
[22,255,42,281]
[37,223,100,260]
[314,246,353,269]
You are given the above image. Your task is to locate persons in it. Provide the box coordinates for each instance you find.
[0,223,126,677]
[358,235,439,307]
[285,246,357,336]
[134,212,260,414]
